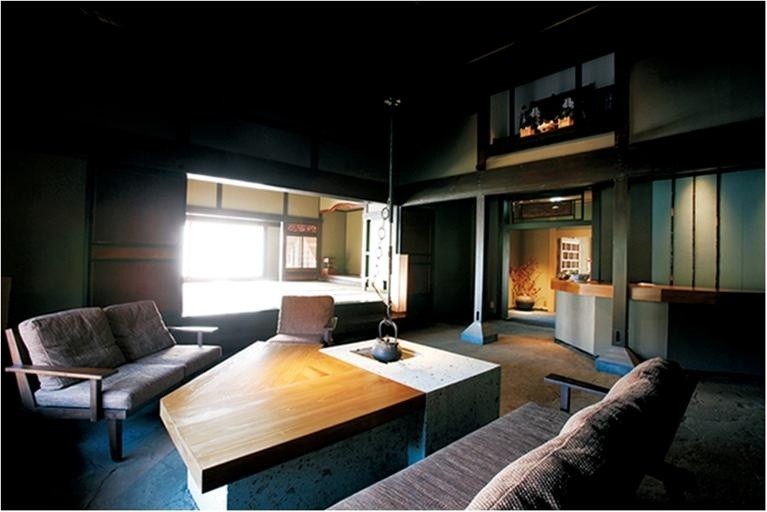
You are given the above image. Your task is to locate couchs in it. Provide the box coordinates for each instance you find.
[326,357,685,510]
[5,300,222,463]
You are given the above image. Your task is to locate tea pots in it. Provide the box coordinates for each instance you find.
[370,319,403,363]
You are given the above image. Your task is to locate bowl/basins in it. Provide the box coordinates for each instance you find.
[571,274,590,283]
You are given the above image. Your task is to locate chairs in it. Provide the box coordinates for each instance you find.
[267,294,336,345]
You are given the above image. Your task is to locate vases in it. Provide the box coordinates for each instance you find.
[516,292,534,310]
